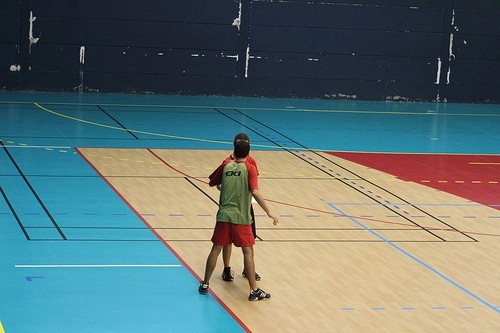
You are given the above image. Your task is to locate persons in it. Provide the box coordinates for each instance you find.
[197,140,278,302]
[214,132,264,281]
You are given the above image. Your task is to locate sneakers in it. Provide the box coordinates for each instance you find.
[198,281,209,295]
[249,288,271,300]
[222,267,234,281]
[242,268,261,280]
